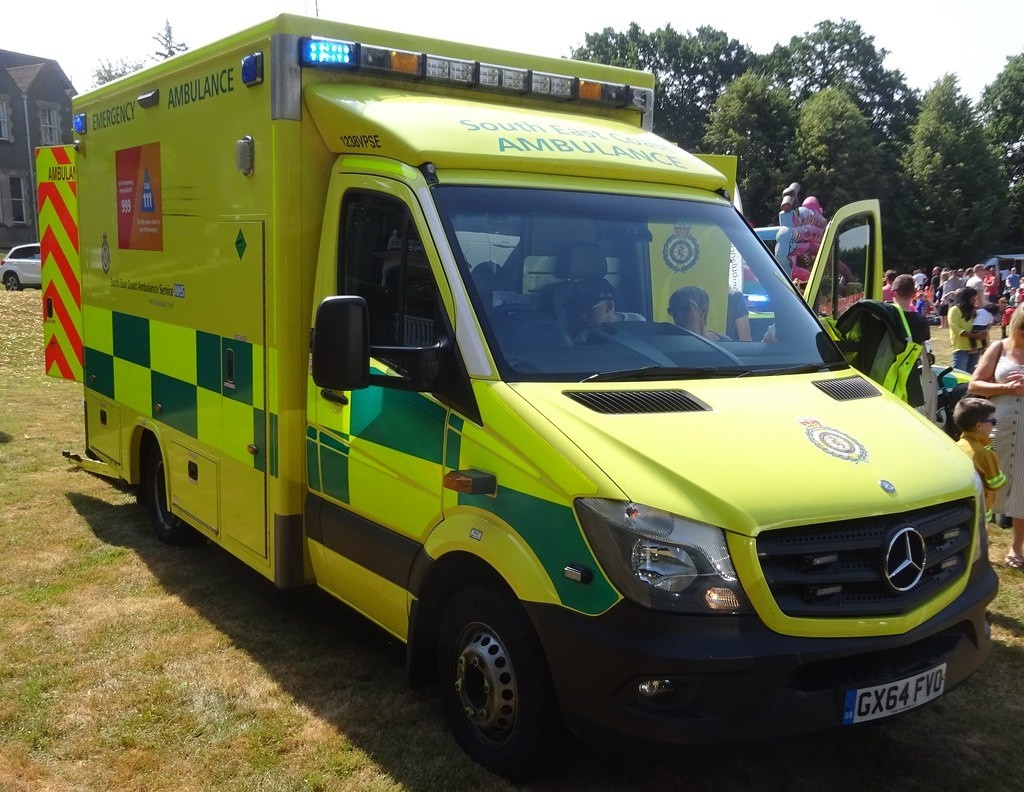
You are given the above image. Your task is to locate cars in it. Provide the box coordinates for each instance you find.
[928,352,973,441]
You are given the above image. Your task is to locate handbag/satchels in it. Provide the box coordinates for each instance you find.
[884,302,925,408]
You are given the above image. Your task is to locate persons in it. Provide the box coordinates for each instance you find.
[968,302,998,355]
[570,276,647,347]
[727,287,752,343]
[888,273,932,360]
[967,301,1024,569]
[667,286,733,342]
[879,263,1024,342]
[947,287,992,375]
[953,397,1006,523]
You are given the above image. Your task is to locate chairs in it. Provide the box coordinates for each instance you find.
[544,241,622,330]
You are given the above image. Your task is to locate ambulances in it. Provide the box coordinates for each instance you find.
[33,13,999,779]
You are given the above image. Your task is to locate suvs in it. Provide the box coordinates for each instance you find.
[0,242,42,292]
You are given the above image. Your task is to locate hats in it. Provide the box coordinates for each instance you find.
[560,276,617,313]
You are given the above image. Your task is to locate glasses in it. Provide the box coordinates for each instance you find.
[977,418,998,426]
[667,297,701,316]
[577,299,611,313]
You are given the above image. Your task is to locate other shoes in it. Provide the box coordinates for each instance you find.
[1005,547,1024,568]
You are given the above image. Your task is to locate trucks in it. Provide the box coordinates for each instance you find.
[977,254,1024,283]
